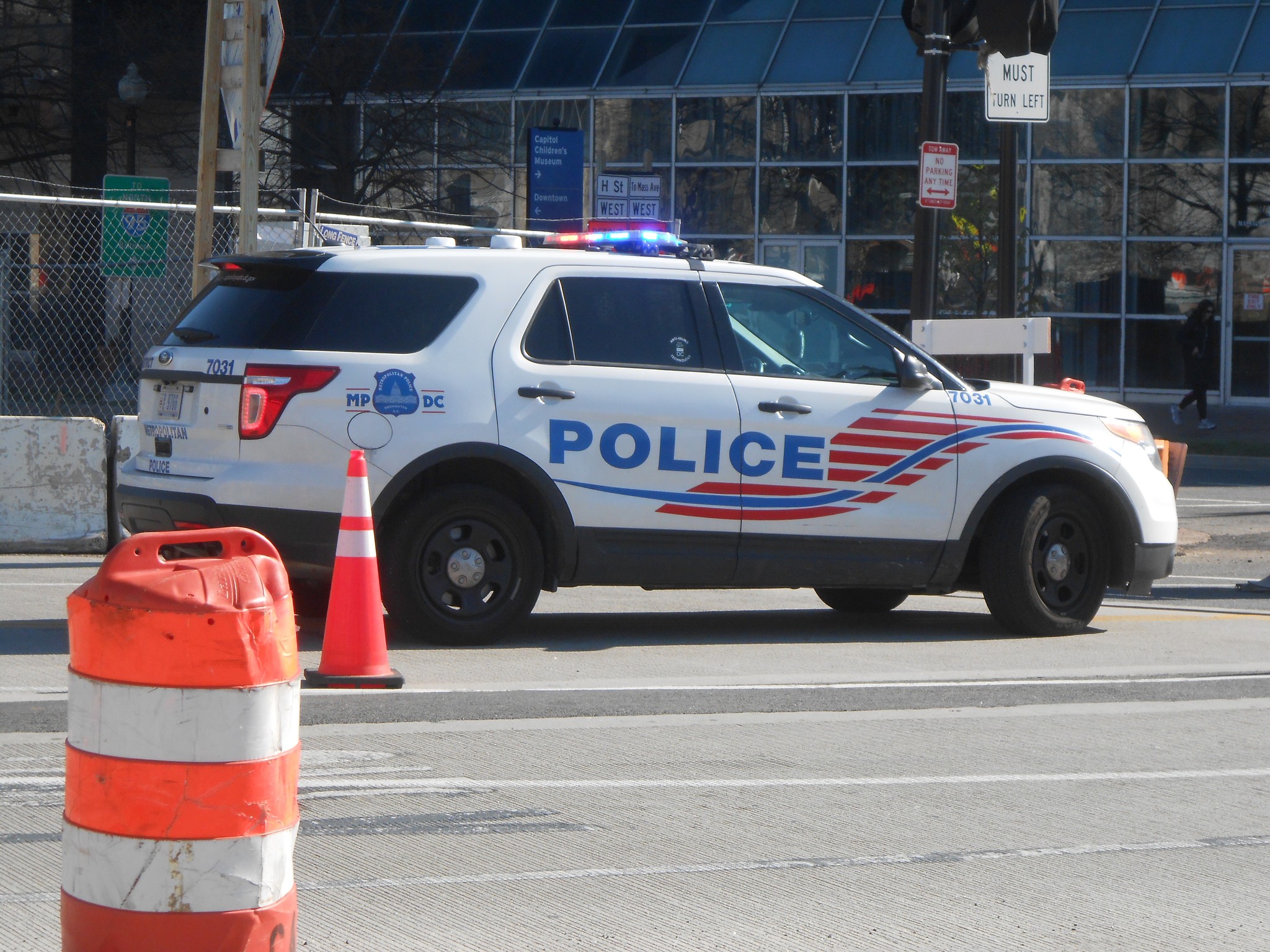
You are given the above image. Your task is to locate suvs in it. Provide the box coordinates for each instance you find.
[113,229,1181,649]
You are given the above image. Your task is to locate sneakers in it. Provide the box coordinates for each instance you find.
[1199,419,1217,429]
[1171,405,1182,425]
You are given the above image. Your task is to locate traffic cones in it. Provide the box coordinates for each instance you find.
[296,446,407,691]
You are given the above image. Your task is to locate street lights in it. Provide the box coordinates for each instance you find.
[114,61,148,413]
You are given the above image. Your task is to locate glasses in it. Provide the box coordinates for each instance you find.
[1206,310,1216,314]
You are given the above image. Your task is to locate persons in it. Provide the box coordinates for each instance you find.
[1172,299,1221,430]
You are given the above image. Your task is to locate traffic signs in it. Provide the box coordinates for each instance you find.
[526,129,585,248]
[921,142,959,212]
